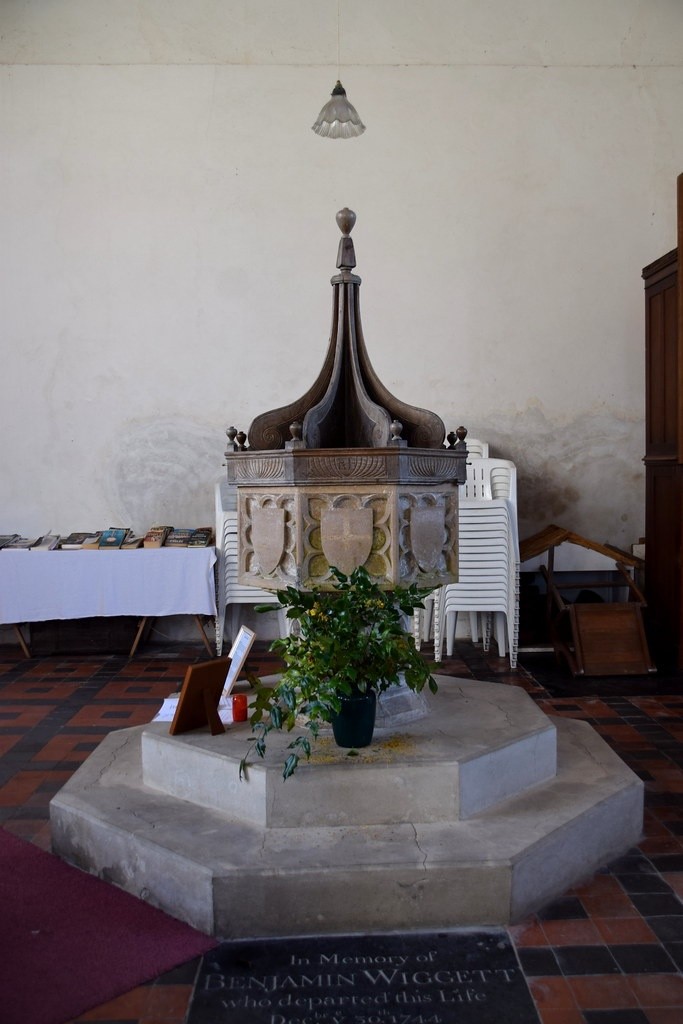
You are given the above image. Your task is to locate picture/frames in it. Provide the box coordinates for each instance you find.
[221,625,256,697]
[169,656,232,735]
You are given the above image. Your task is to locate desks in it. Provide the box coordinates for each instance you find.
[0,543,219,657]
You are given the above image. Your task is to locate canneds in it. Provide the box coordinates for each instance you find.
[232,694,248,722]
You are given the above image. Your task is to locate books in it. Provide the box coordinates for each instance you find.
[1,523,215,551]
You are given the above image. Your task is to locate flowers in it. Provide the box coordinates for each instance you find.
[236,563,440,777]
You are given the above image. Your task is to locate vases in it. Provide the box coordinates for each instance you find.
[328,677,378,748]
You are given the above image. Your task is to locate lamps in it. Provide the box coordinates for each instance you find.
[312,0,366,138]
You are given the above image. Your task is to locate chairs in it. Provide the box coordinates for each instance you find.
[423,437,521,669]
